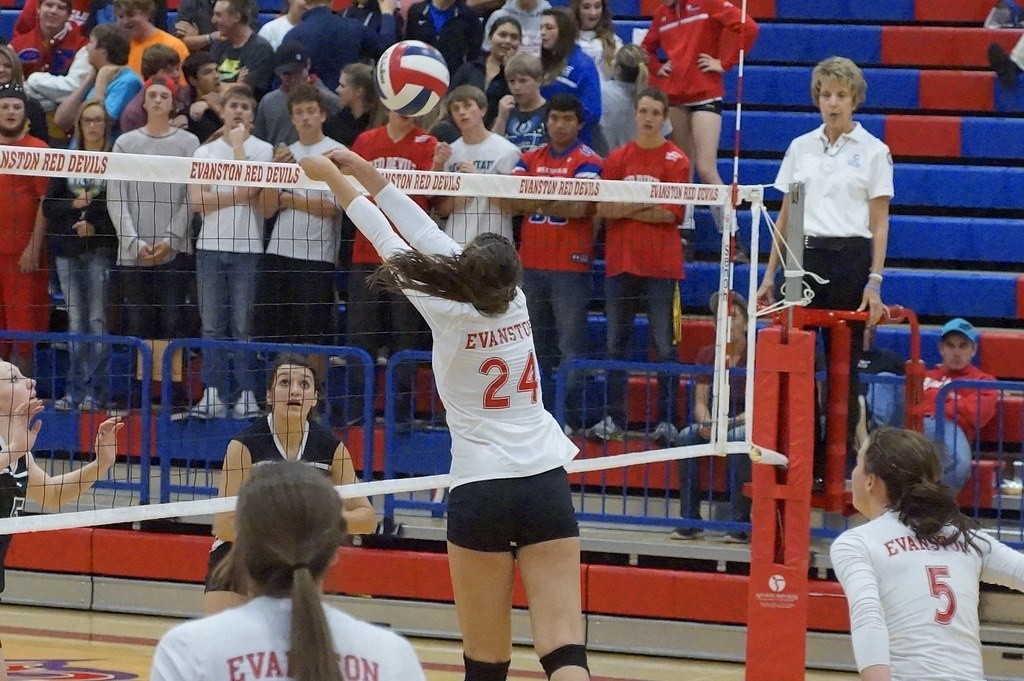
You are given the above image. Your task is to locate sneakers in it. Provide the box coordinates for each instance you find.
[723,532,750,543]
[671,528,704,539]
[233,390,264,418]
[590,415,624,441]
[654,422,678,443]
[190,386,227,419]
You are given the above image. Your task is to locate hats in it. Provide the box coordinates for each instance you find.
[144,74,178,118]
[274,40,307,74]
[0,84,27,103]
[710,291,747,313]
[942,317,979,343]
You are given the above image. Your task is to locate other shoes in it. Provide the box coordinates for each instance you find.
[55,398,74,410]
[78,399,99,412]
[988,43,1019,89]
[732,247,748,264]
[347,397,450,433]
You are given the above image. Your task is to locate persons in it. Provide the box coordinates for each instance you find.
[0,360,124,681]
[297,147,593,681]
[865,318,997,496]
[202,353,376,621]
[149,459,425,681]
[755,57,895,491]
[828,426,1024,681]
[0,0,674,436]
[639,0,759,264]
[988,34,1024,89]
[584,88,691,445]
[672,292,762,544]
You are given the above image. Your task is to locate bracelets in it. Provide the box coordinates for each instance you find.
[867,273,883,282]
[864,284,881,293]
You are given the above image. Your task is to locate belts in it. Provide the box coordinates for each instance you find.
[803,235,867,249]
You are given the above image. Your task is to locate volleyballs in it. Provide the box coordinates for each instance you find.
[373,39,451,117]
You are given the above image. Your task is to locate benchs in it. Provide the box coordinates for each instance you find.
[0,0,1024,511]
